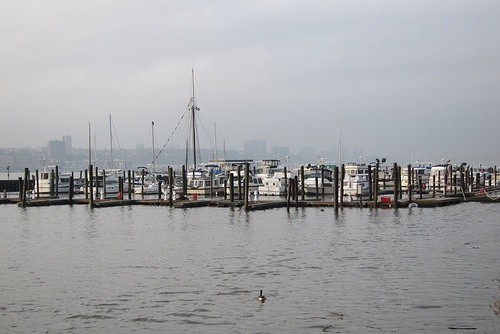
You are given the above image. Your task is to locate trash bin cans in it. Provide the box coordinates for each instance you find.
[193,192,198,202]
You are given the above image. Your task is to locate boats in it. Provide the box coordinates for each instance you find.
[34,158,500,200]
